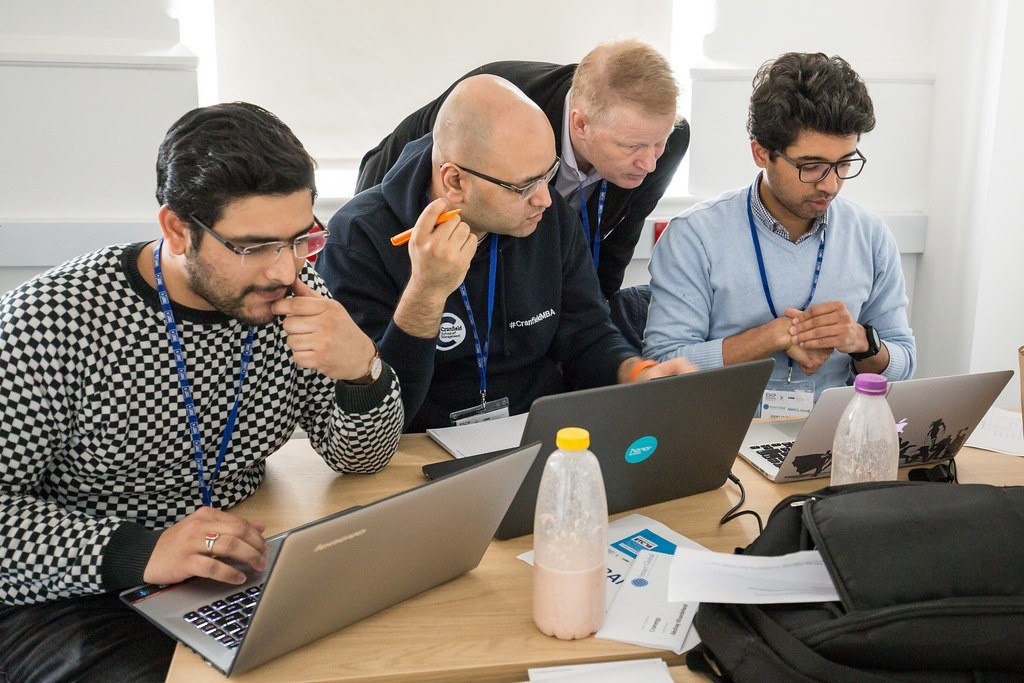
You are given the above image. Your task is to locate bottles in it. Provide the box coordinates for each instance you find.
[532,426,609,640]
[830,374,900,487]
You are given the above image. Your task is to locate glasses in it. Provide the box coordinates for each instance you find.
[190,214,330,268]
[440,156,561,201]
[774,148,867,183]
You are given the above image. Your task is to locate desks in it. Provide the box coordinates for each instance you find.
[165,414,1024,683]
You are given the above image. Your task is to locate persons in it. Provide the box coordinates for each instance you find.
[314,73,700,434]
[0,102,406,683]
[641,52,917,418]
[354,38,691,303]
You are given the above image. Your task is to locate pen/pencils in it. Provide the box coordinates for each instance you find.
[391,208,463,246]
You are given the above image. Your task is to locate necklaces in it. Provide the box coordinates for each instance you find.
[580,179,607,273]
[154,237,254,508]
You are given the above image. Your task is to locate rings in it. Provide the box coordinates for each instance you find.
[205,533,220,555]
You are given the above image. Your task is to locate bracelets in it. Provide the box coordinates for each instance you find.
[628,360,657,384]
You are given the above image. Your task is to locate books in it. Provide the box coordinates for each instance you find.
[426,412,529,459]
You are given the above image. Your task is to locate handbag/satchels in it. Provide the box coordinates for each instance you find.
[685,480,1024,683]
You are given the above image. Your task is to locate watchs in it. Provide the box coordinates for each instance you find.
[849,322,881,362]
[343,338,382,385]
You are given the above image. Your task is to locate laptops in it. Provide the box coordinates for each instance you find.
[120,440,542,679]
[423,357,777,542]
[737,370,1015,482]
